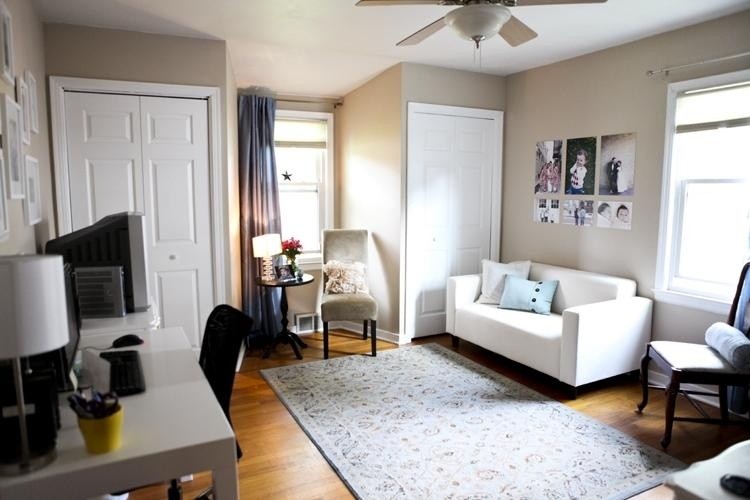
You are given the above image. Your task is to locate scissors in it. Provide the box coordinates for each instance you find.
[97,391,118,419]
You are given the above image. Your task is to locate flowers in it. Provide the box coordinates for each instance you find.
[270,238,304,274]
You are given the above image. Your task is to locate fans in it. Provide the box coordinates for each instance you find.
[350,1,606,46]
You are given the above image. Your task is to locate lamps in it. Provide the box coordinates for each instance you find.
[250,233,282,281]
[445,5,511,47]
[2,254,73,474]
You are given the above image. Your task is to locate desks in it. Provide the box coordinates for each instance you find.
[254,272,315,361]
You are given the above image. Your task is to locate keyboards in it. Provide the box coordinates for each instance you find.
[99,350,145,398]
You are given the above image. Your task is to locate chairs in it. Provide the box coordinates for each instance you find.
[321,228,378,359]
[636,262,750,448]
[110,303,256,500]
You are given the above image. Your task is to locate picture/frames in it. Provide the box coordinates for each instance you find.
[0,8,44,234]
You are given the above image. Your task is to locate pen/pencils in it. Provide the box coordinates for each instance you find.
[67,389,102,419]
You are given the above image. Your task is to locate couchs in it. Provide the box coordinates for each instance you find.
[444,260,654,400]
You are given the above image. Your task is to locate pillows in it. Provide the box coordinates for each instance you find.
[704,320,750,373]
[474,256,559,317]
[321,258,370,295]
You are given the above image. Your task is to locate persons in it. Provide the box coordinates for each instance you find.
[278,265,294,280]
[539,149,630,230]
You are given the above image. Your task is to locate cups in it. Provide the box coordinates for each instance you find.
[76,408,124,454]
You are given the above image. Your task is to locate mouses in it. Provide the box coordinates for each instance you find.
[112,334,144,348]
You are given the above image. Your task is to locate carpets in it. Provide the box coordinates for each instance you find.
[257,340,690,499]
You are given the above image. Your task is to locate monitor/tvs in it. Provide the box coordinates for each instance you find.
[60,263,81,373]
[45,211,150,312]
